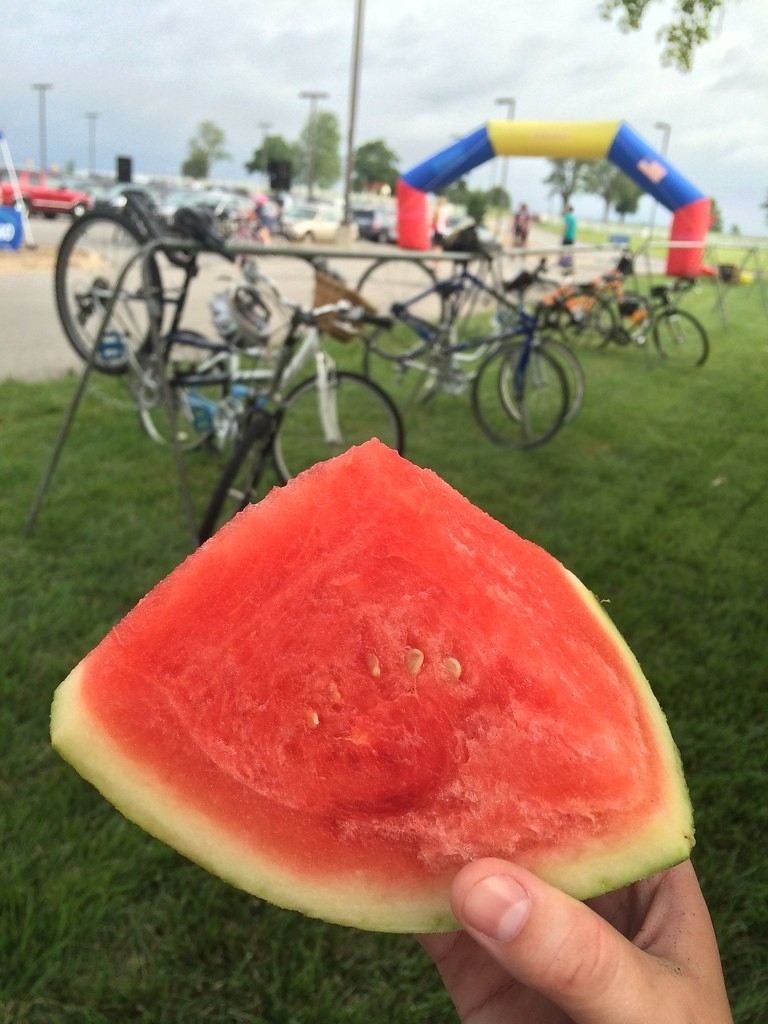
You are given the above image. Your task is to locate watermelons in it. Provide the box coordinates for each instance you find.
[49,437,694,933]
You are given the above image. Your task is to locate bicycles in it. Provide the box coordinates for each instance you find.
[54,188,715,489]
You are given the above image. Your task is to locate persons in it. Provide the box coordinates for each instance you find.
[511,204,531,261]
[245,192,273,247]
[410,856,733,1024]
[561,206,578,269]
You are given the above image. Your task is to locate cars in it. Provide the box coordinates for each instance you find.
[61,166,502,254]
[0,165,93,223]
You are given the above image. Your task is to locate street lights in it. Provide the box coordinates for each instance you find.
[650,119,675,231]
[497,97,518,194]
[301,88,331,196]
[34,80,49,176]
[86,110,102,176]
